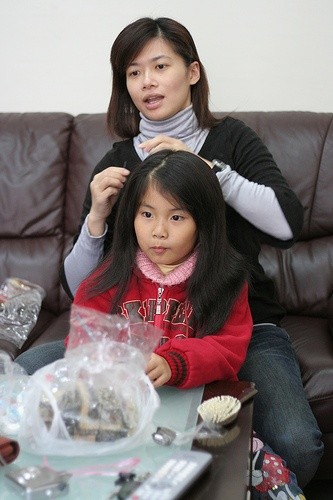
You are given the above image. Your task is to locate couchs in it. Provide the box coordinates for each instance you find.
[0,112,333,500]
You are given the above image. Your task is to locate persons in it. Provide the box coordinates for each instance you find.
[13,18,325,487]
[63,149,306,500]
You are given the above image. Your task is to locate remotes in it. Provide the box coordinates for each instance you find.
[125,450,212,500]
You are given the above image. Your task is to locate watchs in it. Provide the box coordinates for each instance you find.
[211,159,226,174]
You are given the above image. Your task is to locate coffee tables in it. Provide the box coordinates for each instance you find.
[2,380,256,500]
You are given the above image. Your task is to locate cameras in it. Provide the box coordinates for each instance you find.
[4,464,69,500]
